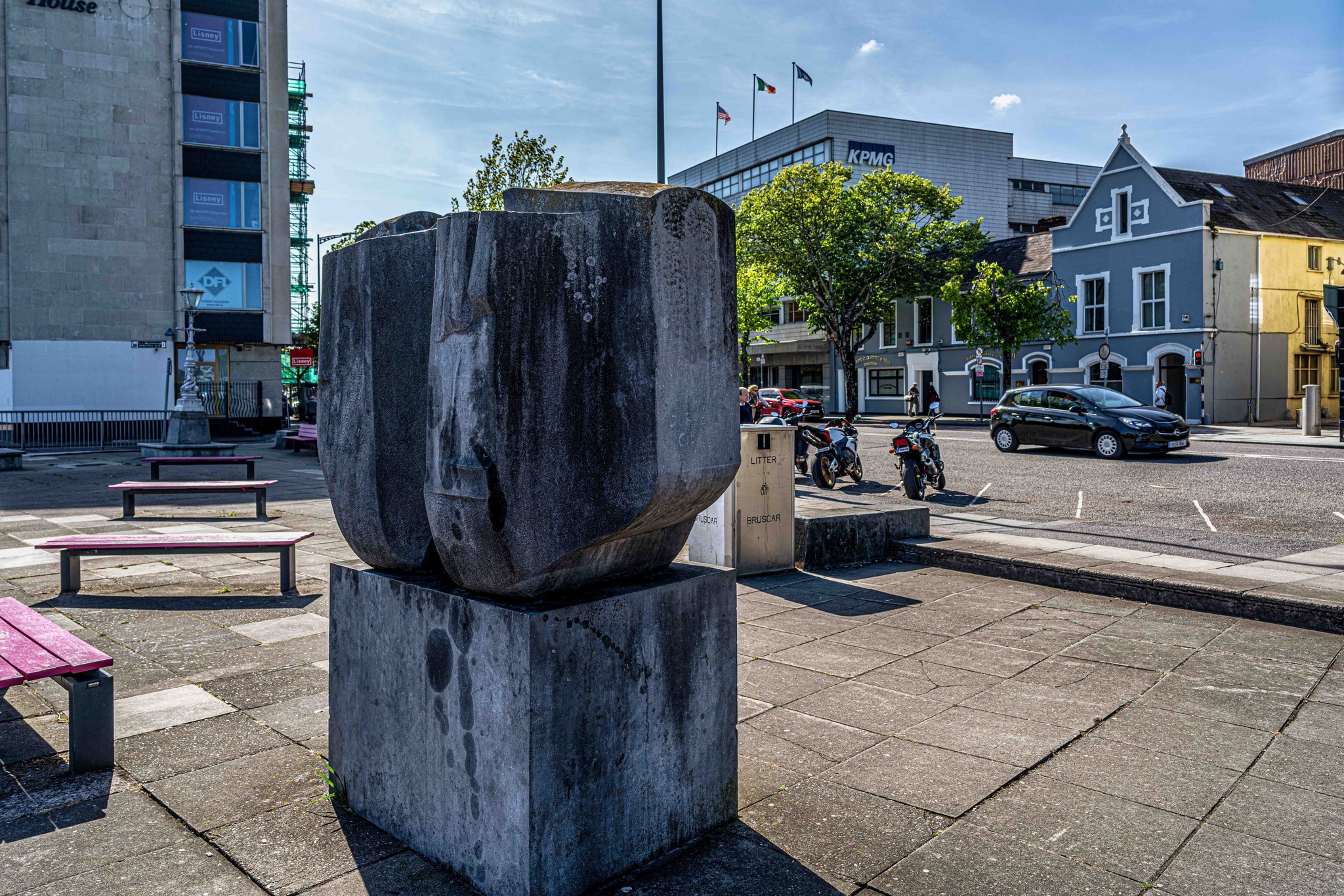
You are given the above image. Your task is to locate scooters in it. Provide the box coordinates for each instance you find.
[758,400,812,474]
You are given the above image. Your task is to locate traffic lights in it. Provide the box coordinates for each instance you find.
[1335,340,1339,346]
[1194,350,1203,366]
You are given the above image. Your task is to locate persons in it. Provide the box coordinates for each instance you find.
[747,385,771,424]
[739,387,754,425]
[927,385,939,417]
[1154,381,1166,410]
[908,382,920,417]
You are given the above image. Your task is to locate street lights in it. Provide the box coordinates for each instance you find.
[174,280,207,412]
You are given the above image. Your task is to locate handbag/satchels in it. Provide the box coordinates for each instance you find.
[904,394,913,402]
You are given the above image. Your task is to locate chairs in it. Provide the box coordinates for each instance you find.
[1048,391,1057,409]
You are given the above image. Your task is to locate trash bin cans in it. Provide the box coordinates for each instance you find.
[1302,384,1321,436]
[687,425,796,575]
[1296,408,1302,429]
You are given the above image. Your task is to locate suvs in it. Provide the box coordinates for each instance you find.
[757,388,825,423]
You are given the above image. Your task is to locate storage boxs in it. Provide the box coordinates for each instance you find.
[758,416,783,425]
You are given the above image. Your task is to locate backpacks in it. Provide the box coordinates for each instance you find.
[1157,388,1173,405]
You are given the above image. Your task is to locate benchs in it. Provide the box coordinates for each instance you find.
[34,531,315,593]
[0,596,114,770]
[141,456,263,480]
[282,424,318,456]
[0,448,27,471]
[109,480,278,518]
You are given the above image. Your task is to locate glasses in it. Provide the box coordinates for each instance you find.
[1066,395,1073,398]
[753,390,758,392]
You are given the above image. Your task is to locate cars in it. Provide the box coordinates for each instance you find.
[990,384,1190,459]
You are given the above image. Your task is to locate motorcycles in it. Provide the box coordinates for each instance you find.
[801,414,863,489]
[888,401,946,501]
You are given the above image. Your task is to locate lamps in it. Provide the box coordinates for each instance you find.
[1326,257,1344,274]
